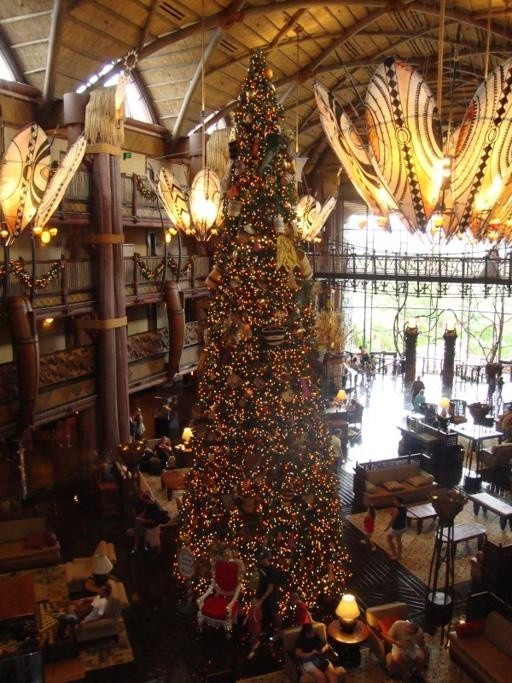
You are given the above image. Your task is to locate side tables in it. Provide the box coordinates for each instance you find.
[328,616,370,670]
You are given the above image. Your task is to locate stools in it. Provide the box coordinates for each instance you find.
[0,574,41,631]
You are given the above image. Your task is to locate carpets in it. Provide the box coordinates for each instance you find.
[234,609,479,683]
[1,560,136,681]
[447,424,508,472]
[344,497,512,590]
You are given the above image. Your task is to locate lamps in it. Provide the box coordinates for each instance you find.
[282,28,335,245]
[335,593,361,633]
[338,391,350,405]
[181,427,194,444]
[311,1,511,245]
[313,0,512,242]
[2,121,86,245]
[155,2,227,243]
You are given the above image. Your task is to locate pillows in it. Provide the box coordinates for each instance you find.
[383,481,404,491]
[407,475,433,487]
[24,529,46,548]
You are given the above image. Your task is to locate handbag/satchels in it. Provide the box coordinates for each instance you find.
[307,654,329,671]
[159,511,168,524]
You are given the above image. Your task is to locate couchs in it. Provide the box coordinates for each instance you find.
[470,538,512,588]
[0,647,45,682]
[0,517,61,569]
[280,621,340,682]
[448,588,512,683]
[352,450,437,510]
[108,460,165,555]
[364,601,430,678]
[143,438,175,470]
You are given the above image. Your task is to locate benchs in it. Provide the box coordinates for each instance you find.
[468,492,512,531]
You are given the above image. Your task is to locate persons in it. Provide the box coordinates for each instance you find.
[475,243,502,279]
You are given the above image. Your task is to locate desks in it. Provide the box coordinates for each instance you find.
[174,442,196,465]
[405,500,487,561]
[328,616,370,670]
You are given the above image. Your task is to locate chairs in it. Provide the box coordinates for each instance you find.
[397,399,512,473]
[145,437,175,469]
[320,395,363,443]
[95,460,178,555]
[364,601,430,678]
[417,399,512,473]
[65,540,130,652]
[280,621,340,682]
[197,558,244,637]
